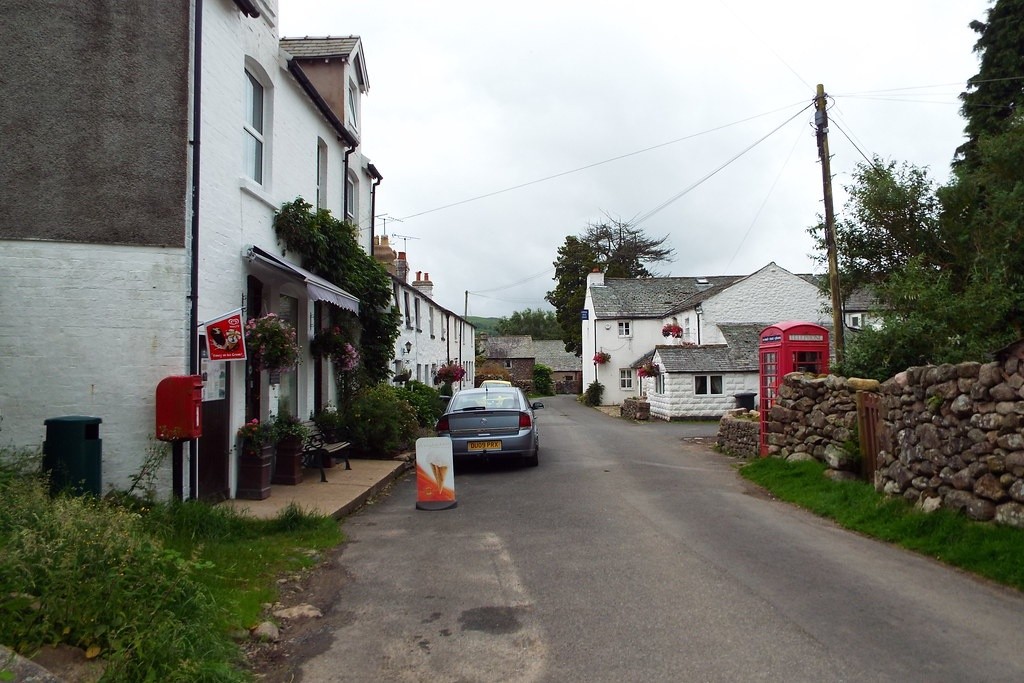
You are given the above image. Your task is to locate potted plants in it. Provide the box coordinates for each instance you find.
[273,413,306,485]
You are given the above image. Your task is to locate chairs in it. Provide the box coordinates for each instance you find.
[503,398,513,408]
[464,400,478,407]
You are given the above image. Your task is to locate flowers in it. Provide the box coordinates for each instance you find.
[313,327,360,371]
[637,361,659,377]
[246,312,302,372]
[439,364,466,384]
[594,352,611,364]
[662,323,683,338]
[238,418,280,449]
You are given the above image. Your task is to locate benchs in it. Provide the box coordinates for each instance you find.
[299,419,353,484]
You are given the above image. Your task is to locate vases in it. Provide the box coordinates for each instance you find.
[237,445,271,500]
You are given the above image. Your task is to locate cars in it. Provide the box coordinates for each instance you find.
[475,380,514,407]
[435,387,545,467]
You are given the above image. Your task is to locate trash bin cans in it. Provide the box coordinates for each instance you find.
[732,392,757,412]
[40,416,104,509]
